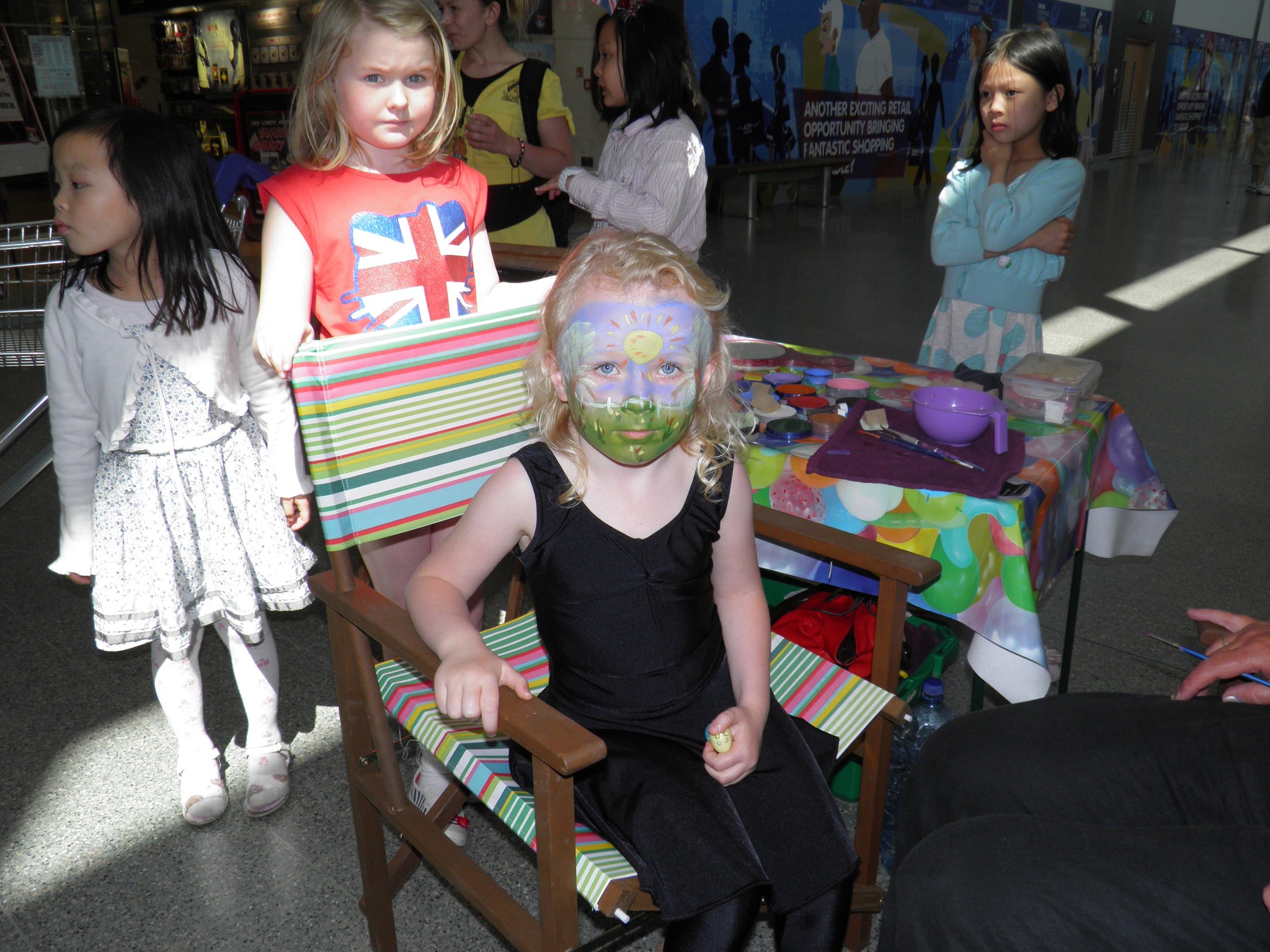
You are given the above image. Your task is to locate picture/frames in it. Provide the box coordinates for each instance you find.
[22,31,86,99]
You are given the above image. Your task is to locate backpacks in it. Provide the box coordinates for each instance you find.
[440,48,576,248]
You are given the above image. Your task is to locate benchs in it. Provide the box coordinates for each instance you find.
[706,158,847,218]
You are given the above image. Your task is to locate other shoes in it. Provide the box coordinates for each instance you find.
[409,764,471,847]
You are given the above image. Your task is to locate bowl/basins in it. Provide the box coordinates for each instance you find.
[911,386,1008,454]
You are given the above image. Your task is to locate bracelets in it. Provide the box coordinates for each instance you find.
[509,137,525,168]
[998,250,1012,269]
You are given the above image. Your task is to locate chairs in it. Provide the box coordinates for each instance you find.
[292,301,941,952]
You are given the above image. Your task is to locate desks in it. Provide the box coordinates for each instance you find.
[722,335,1178,710]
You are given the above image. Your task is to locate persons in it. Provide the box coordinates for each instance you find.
[193,18,210,94]
[916,23,1086,374]
[535,8,708,262]
[439,0,578,247]
[1245,69,1270,195]
[44,98,316,827]
[251,0,557,849]
[879,609,1269,952]
[405,230,864,952]
[228,19,246,93]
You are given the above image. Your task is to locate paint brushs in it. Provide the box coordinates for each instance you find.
[879,424,986,472]
[857,430,960,465]
[1143,631,1270,689]
[878,431,975,470]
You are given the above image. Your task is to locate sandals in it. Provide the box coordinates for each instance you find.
[177,747,228,825]
[242,742,291,818]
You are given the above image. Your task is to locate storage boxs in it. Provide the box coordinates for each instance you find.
[1001,352,1103,426]
